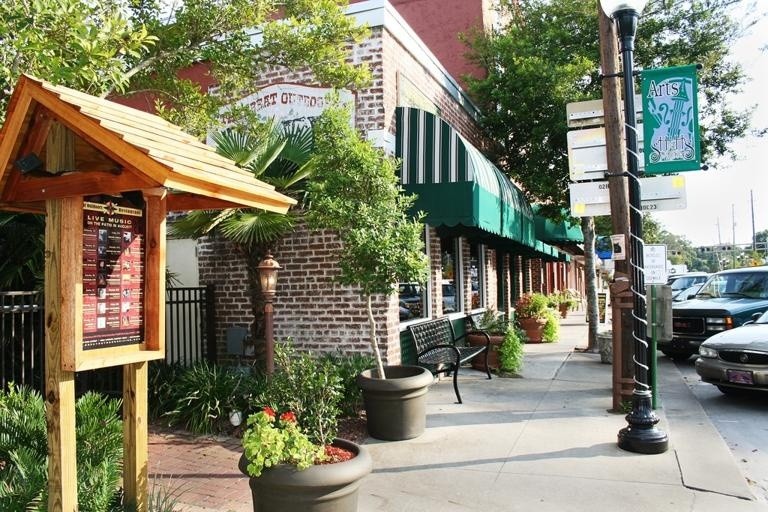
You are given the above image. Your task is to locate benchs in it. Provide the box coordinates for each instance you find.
[402,317,491,405]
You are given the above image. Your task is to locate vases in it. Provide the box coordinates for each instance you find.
[240,435,371,512]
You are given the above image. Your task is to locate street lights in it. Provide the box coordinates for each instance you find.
[593,0,671,454]
[254,248,282,413]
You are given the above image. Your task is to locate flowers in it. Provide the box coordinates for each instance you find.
[238,341,346,476]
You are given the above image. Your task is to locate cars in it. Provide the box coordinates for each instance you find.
[656,261,768,400]
[398,278,479,322]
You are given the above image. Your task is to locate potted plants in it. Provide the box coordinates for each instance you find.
[466,302,520,382]
[510,291,556,347]
[336,219,433,442]
[545,286,575,322]
